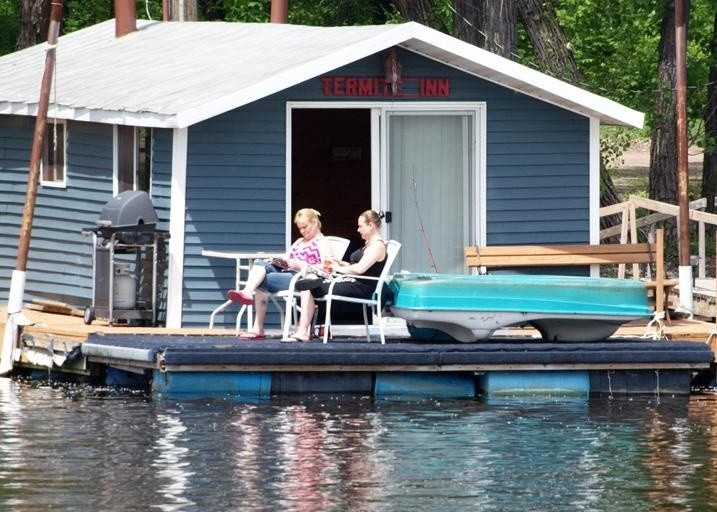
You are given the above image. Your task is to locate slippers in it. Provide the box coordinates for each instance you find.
[280,336,313,343]
[239,332,266,340]
[295,277,323,291]
[227,290,254,305]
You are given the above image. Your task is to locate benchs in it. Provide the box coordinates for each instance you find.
[465,242,680,325]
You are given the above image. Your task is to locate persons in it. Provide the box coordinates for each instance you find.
[226,208,387,343]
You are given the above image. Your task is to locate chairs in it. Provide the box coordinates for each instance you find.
[268,235,403,345]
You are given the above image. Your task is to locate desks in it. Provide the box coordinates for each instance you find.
[201,250,289,336]
